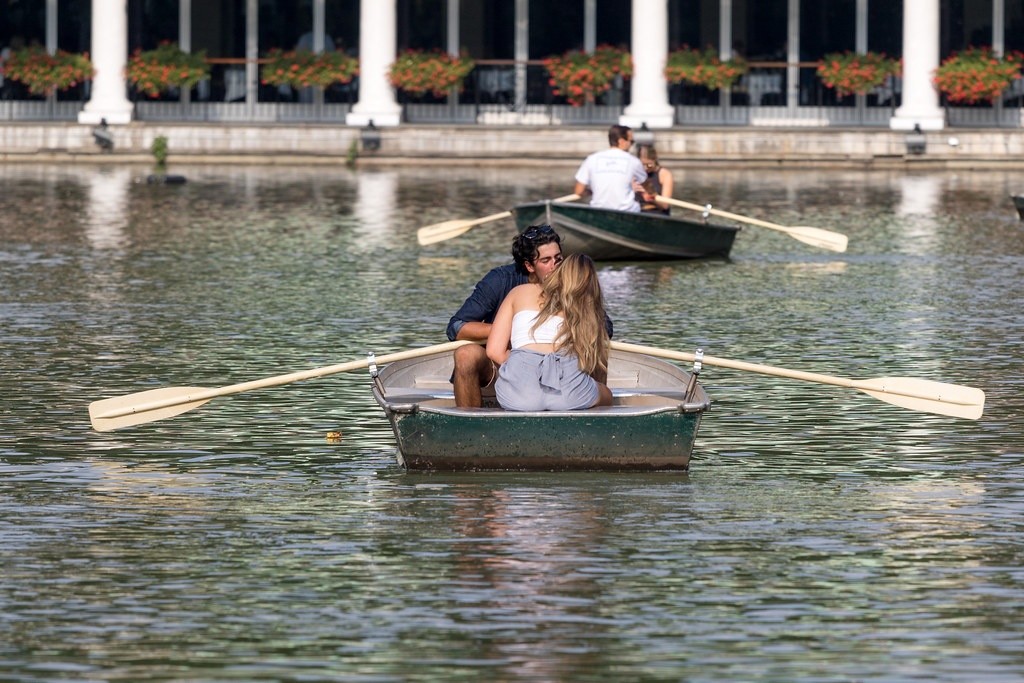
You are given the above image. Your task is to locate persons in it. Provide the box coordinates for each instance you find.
[634,146,673,217]
[574,125,648,215]
[446,223,614,412]
[486,252,614,411]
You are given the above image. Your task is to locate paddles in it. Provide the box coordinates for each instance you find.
[606,340,986,422]
[87,340,489,434]
[417,194,582,246]
[654,193,849,254]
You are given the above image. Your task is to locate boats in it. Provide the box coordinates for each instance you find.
[510,201,741,262]
[368,347,711,471]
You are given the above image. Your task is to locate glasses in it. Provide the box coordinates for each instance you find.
[554,257,562,266]
[523,225,552,239]
[643,163,654,168]
[624,138,635,145]
